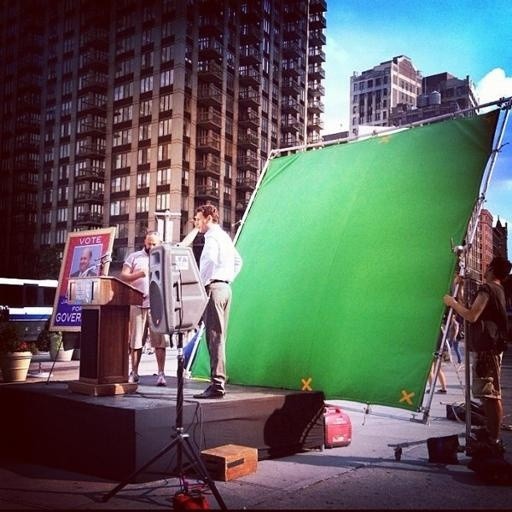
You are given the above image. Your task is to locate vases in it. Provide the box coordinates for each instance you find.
[49,336,74,361]
[0,351,32,382]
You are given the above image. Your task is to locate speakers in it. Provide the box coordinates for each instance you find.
[147,244,210,333]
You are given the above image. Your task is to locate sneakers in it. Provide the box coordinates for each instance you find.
[156,374,167,386]
[128,374,140,385]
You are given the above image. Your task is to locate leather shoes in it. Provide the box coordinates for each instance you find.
[193,386,223,399]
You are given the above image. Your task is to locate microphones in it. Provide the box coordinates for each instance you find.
[95,251,113,267]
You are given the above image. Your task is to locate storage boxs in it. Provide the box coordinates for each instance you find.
[200,444,258,482]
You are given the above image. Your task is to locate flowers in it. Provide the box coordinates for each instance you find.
[0,336,39,356]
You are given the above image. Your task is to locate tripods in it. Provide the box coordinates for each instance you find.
[102,339,231,512]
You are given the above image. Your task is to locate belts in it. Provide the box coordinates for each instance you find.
[211,280,228,283]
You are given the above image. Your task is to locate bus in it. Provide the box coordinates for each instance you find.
[0,277,59,352]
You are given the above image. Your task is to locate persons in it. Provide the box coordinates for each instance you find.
[446,312,462,364]
[441,254,512,456]
[71,247,99,277]
[192,204,243,399]
[120,232,172,388]
[427,326,449,393]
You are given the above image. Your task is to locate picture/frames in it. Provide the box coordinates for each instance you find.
[48,227,116,333]
